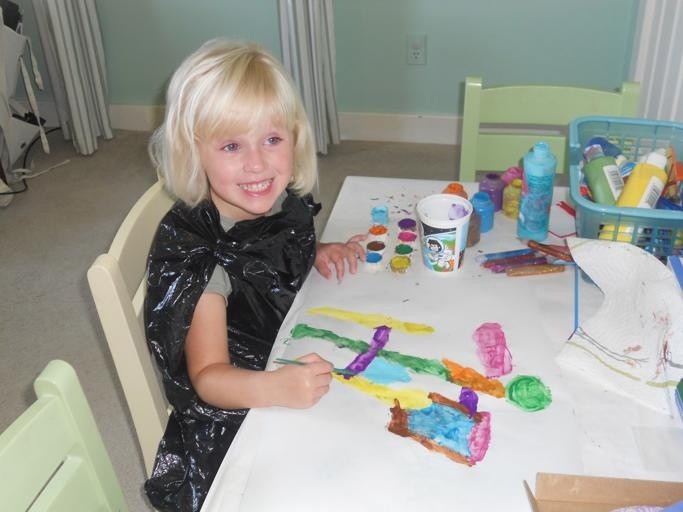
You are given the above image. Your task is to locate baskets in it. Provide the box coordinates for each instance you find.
[567,116,683,284]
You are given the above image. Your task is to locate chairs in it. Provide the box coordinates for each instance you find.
[0,359,131,512]
[459,77,642,189]
[88,168,204,478]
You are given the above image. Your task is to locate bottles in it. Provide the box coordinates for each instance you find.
[450,140,557,242]
[579,137,682,247]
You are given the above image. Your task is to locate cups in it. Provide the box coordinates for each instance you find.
[415,193,473,273]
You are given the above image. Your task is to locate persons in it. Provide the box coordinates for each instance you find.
[143,37,367,512]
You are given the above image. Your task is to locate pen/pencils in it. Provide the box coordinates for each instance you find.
[273,358,359,376]
[474,240,574,276]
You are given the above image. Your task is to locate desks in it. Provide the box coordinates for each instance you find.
[198,176,683,512]
